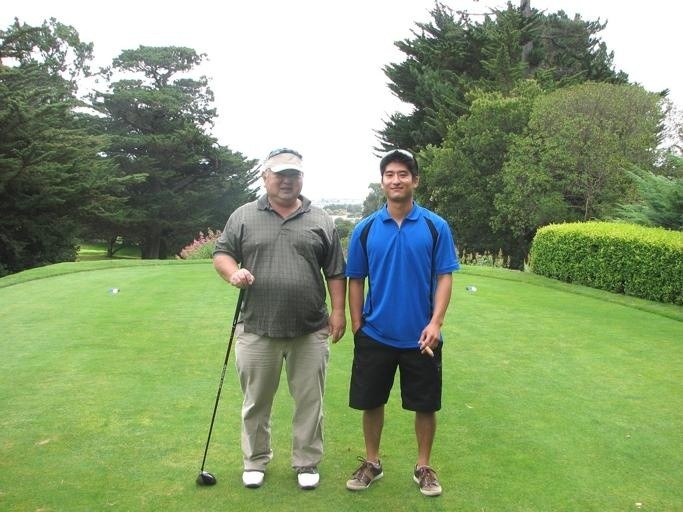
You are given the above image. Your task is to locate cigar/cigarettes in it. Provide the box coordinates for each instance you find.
[421,341,435,359]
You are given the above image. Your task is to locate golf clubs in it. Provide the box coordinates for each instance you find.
[195,288,245,486]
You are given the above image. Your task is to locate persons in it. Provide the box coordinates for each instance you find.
[210,147,348,493]
[341,147,462,497]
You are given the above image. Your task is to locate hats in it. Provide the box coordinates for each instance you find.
[264,148,304,173]
[380,149,417,175]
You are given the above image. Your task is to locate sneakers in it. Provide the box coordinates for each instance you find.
[346,459,383,491]
[242,469,264,488]
[413,463,442,496]
[297,468,320,488]
